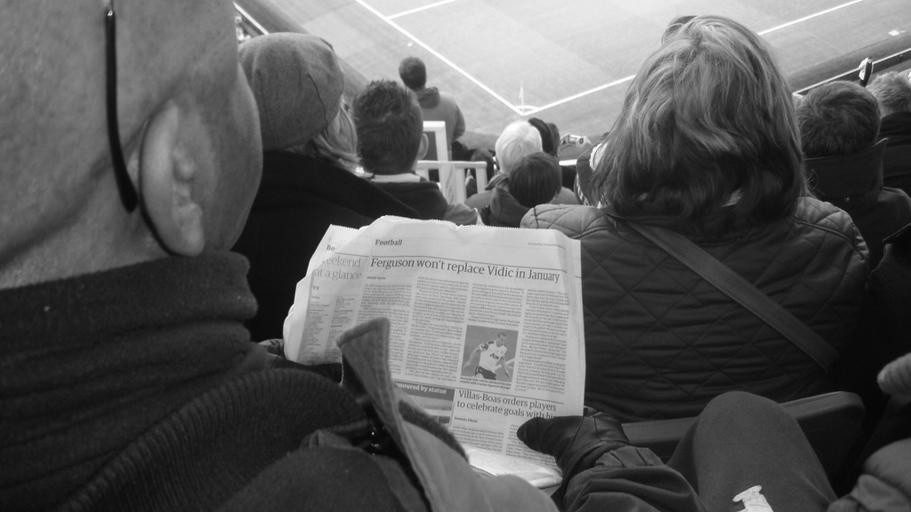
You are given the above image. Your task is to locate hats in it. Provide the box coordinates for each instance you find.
[236,30,345,151]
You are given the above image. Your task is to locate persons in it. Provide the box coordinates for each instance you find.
[517,11,872,424]
[397,55,466,182]
[794,80,911,245]
[463,332,513,382]
[866,72,911,196]
[349,80,486,227]
[665,341,910,511]
[899,69,911,85]
[466,118,582,229]
[229,31,424,341]
[0,0,712,512]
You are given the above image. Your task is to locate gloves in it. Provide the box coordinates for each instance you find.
[517,404,635,484]
